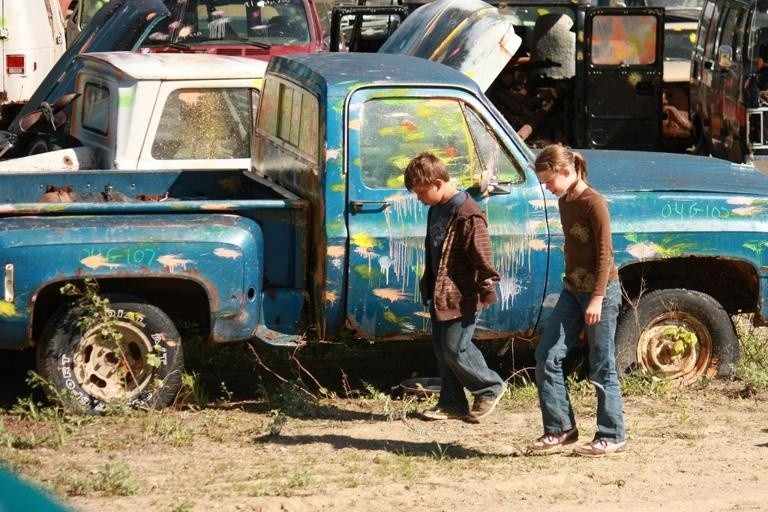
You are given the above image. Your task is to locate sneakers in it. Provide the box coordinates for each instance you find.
[423,383,506,421]
[527,427,627,457]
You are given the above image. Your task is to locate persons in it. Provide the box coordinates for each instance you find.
[529,144,628,458]
[405,153,508,422]
